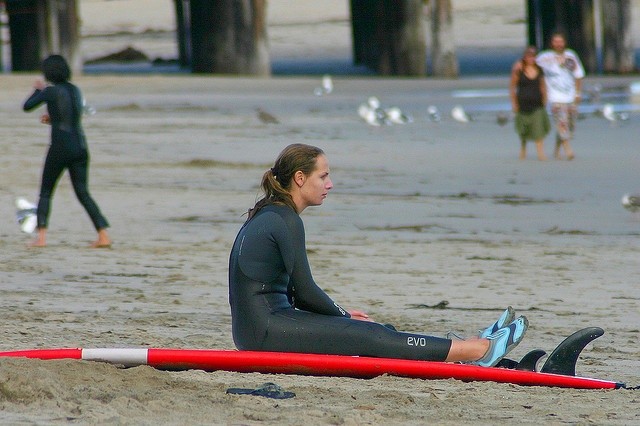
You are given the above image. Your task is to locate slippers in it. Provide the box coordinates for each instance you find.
[227,382,296,399]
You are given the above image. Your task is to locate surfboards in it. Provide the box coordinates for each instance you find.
[1,326,629,412]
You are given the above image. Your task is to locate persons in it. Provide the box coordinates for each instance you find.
[22,55,113,248]
[509,46,551,161]
[512,33,585,162]
[228,143,529,369]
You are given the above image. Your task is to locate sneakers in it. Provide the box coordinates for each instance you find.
[479,306,516,339]
[473,315,530,367]
